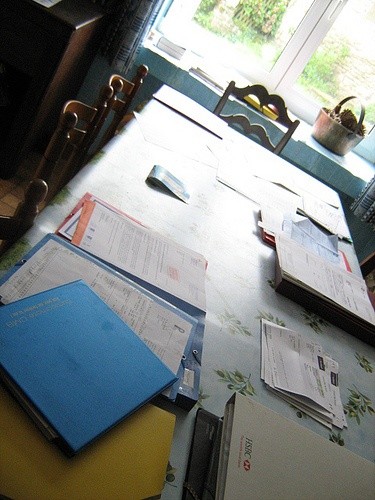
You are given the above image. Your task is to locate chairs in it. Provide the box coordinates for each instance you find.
[213,81,300,155]
[74,62,149,178]
[0,84,114,259]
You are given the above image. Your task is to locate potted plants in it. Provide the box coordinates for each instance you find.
[310,92,366,155]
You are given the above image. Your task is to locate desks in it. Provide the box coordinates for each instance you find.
[0,0,122,178]
[1,83,375,500]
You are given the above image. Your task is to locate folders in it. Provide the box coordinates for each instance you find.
[184,392,375,500]
[0,192,207,453]
[274,247,375,348]
